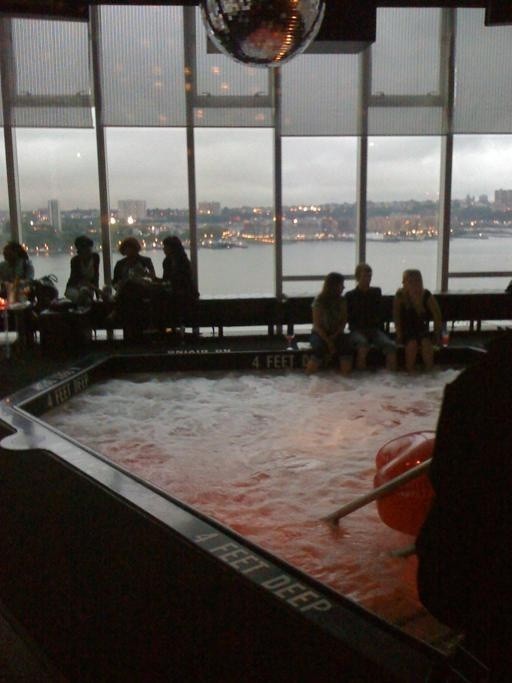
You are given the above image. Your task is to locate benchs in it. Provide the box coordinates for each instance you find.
[284,293,512,339]
[87,298,277,344]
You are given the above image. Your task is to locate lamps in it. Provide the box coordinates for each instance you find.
[202,0,325,67]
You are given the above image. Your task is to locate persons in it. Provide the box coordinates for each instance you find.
[345,264,398,373]
[306,274,352,378]
[412,326,508,628]
[0,240,33,300]
[147,234,193,341]
[105,238,154,340]
[394,270,444,374]
[65,235,103,309]
